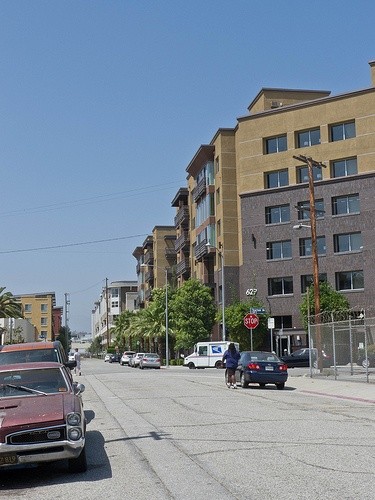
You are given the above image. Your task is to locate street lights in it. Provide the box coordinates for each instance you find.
[140,264,168,367]
[206,243,225,341]
[293,224,312,229]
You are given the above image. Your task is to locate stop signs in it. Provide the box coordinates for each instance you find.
[244,314,259,329]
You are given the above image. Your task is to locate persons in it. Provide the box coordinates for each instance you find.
[74,348,82,376]
[222,343,241,389]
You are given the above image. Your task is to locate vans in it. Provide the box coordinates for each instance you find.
[183,341,239,369]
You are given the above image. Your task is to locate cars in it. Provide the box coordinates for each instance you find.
[281,348,332,369]
[225,351,288,390]
[104,351,160,369]
[0,341,87,472]
[357,351,375,368]
